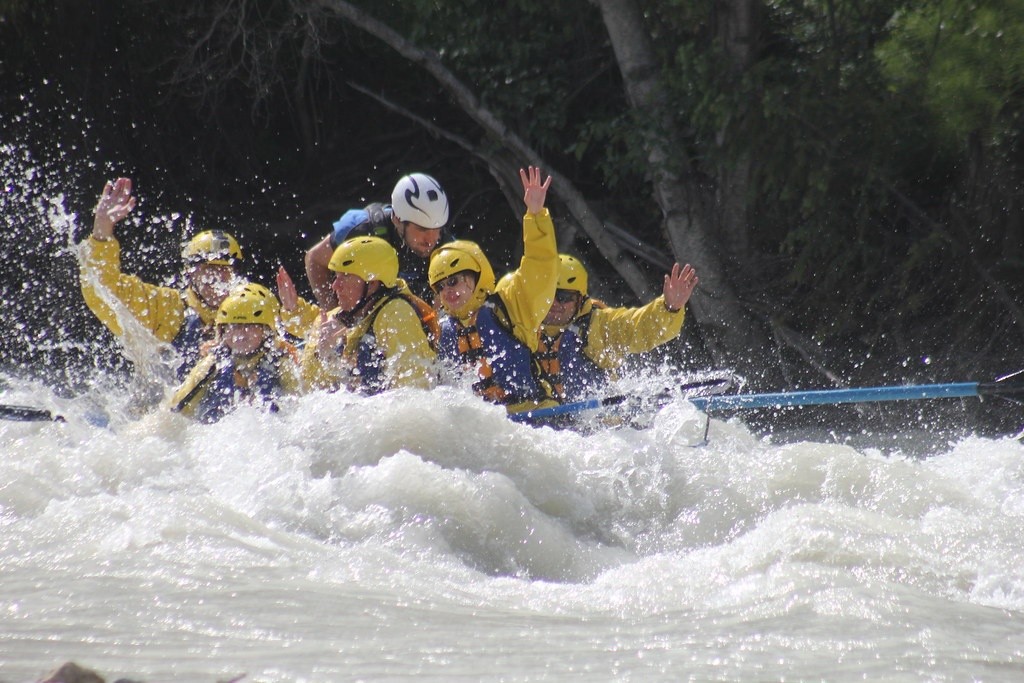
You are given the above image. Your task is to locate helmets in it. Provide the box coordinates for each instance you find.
[428,239,496,320]
[181,229,245,268]
[556,253,588,297]
[215,282,281,341]
[328,235,400,288]
[390,173,450,229]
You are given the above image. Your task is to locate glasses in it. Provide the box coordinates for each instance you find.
[555,292,578,303]
[435,277,458,292]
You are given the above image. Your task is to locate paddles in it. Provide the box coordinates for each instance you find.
[514,377,733,423]
[685,379,1024,412]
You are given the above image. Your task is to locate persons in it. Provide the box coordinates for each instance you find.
[79,166,699,432]
